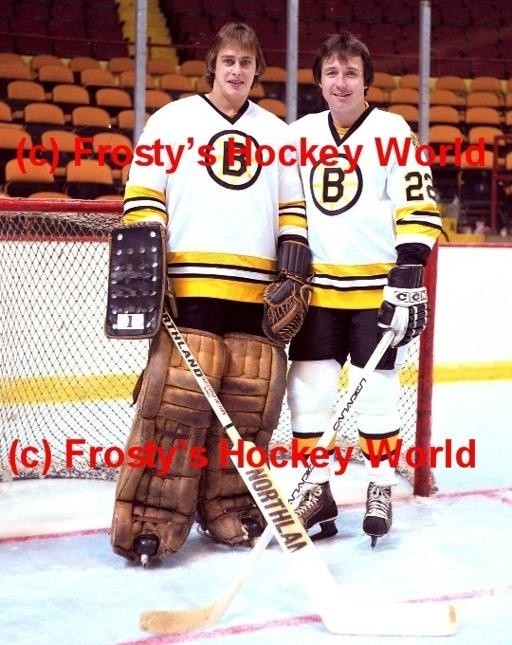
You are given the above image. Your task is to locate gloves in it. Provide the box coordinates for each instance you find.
[379,265,429,349]
[260,240,315,347]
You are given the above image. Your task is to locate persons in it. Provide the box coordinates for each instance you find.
[112,21,311,563]
[280,31,442,535]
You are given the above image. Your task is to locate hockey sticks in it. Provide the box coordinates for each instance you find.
[140,329,390,635]
[162,308,458,635]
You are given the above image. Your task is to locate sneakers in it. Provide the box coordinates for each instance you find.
[133,532,159,557]
[295,482,337,528]
[362,482,392,534]
[238,515,260,536]
